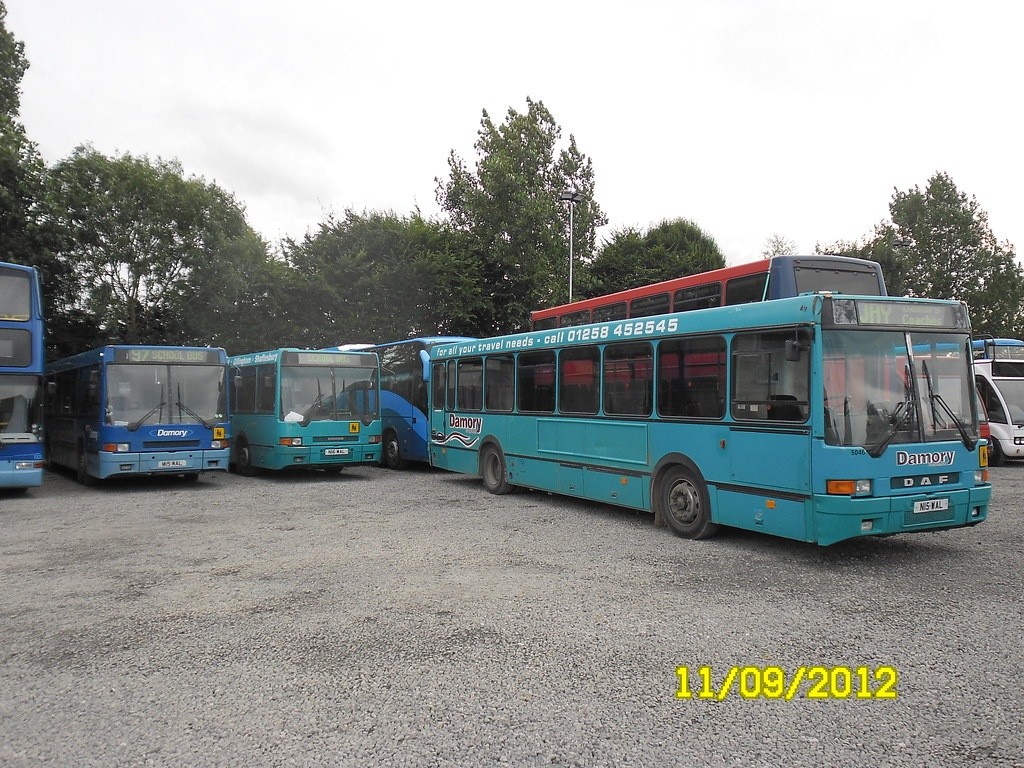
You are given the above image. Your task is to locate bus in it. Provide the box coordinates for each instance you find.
[46,345,234,485]
[894,337,1024,363]
[229,347,383,476]
[822,355,991,467]
[528,253,891,393]
[313,335,478,468]
[426,295,992,548]
[0,258,48,491]
[973,358,1023,466]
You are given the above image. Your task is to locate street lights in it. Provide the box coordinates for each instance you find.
[560,193,586,304]
